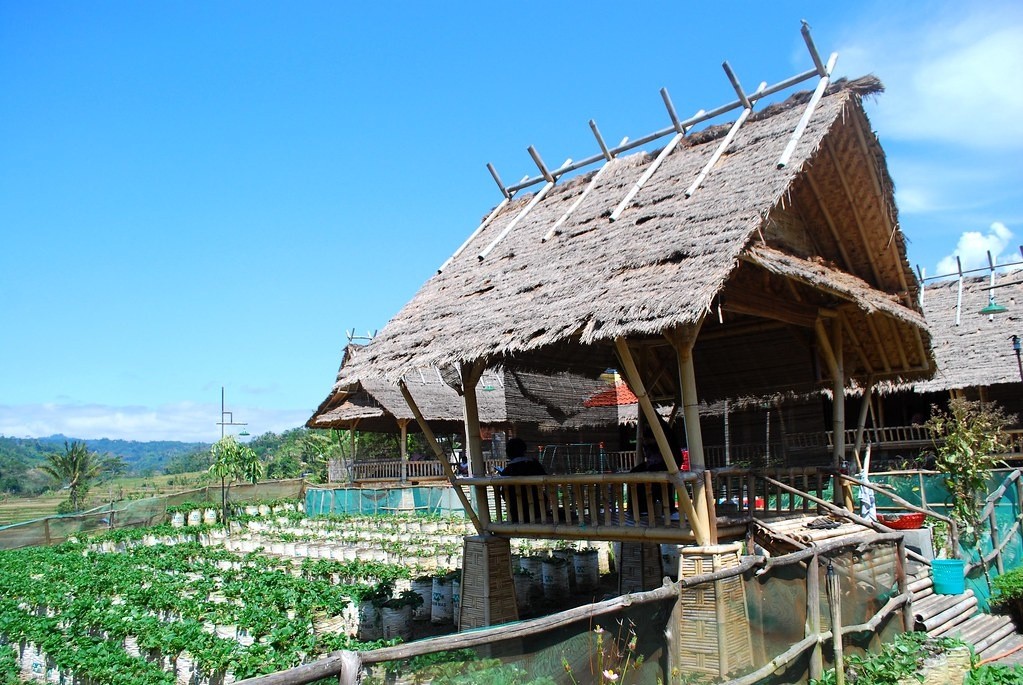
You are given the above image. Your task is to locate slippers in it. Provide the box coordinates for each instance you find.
[802,517,842,529]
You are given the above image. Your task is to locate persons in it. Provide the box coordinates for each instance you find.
[456,456,468,473]
[410,449,425,461]
[502,439,576,523]
[624,440,682,516]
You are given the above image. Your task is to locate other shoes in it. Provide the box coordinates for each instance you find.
[664,511,688,521]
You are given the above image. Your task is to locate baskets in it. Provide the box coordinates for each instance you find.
[876,512,926,528]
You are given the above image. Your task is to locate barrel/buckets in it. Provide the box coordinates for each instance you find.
[931,560,964,594]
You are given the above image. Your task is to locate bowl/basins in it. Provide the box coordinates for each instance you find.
[876,513,927,529]
